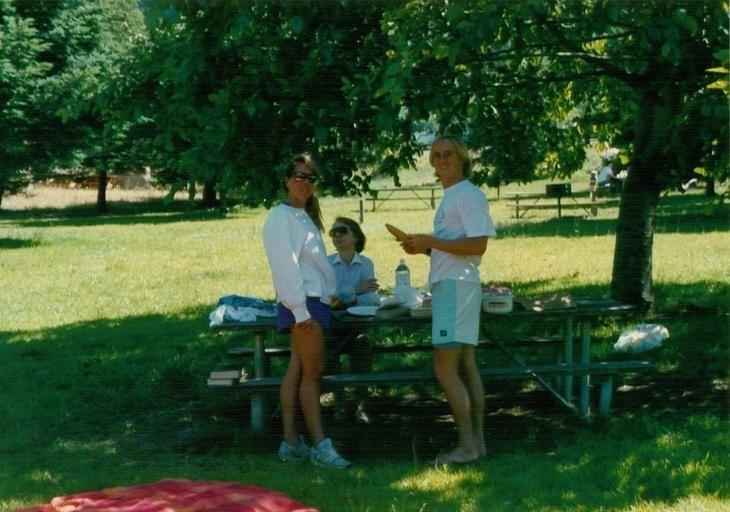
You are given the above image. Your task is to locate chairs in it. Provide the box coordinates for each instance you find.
[598,178,616,197]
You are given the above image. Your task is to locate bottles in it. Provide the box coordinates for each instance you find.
[394,259,410,290]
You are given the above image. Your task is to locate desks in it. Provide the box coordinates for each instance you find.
[212,297,637,435]
[366,186,443,212]
[505,191,597,219]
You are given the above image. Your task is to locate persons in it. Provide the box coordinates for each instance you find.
[261,153,352,473]
[324,216,382,433]
[393,135,500,469]
[597,161,620,195]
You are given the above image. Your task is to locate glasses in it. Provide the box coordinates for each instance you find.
[288,170,318,184]
[328,226,350,237]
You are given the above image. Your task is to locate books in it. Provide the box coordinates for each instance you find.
[207,377,235,386]
[210,363,244,379]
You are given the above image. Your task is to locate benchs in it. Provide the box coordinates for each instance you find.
[207,358,652,435]
[506,202,608,219]
[225,336,597,398]
[365,196,442,211]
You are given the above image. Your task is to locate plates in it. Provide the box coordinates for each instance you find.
[347,306,380,315]
[385,222,408,240]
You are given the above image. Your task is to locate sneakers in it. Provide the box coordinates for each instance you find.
[276,433,312,464]
[307,436,353,470]
[317,392,370,426]
[426,437,489,468]
